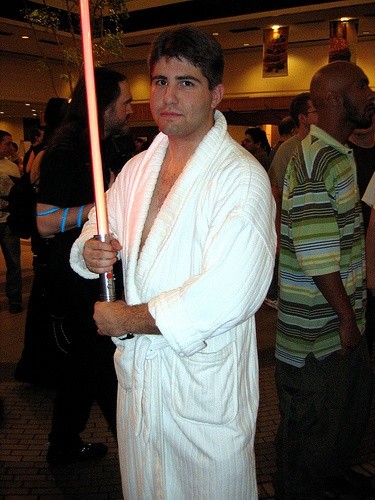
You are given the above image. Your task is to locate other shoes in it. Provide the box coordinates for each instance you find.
[264,298,279,309]
[48,439,106,465]
[326,468,370,495]
[9,303,22,312]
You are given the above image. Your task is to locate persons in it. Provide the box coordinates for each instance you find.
[35,69,135,470]
[0,97,161,388]
[69,27,277,500]
[243,61,375,500]
